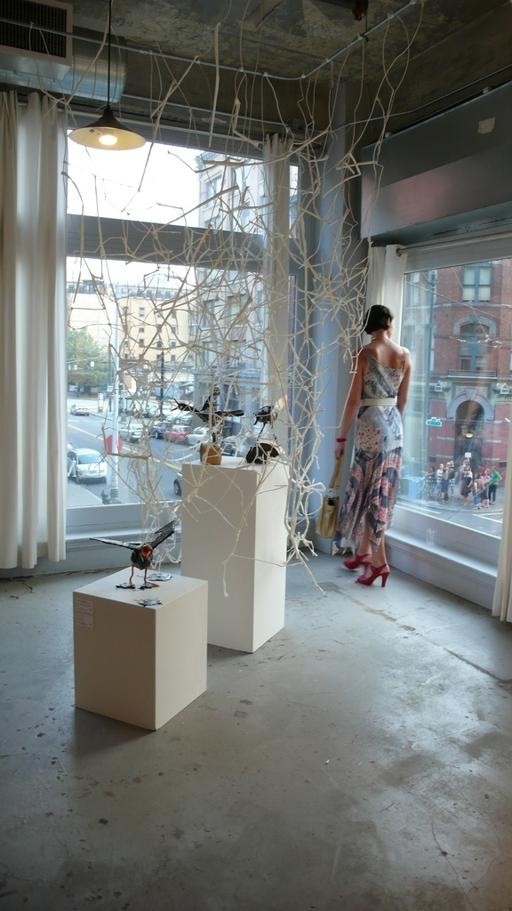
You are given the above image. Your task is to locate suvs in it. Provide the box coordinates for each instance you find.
[68,448,109,485]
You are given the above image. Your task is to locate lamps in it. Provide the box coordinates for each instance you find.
[67,0,147,154]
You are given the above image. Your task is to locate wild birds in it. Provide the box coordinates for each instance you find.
[88,520,176,590]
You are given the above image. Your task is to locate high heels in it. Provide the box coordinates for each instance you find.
[357,564,391,588]
[344,552,372,572]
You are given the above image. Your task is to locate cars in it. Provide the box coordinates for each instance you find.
[174,470,183,497]
[119,420,255,459]
[70,385,85,393]
[70,403,91,417]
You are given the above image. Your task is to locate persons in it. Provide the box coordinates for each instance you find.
[334,304,412,589]
[421,460,504,510]
[66,444,79,479]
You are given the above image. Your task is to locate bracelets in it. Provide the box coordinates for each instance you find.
[336,438,348,444]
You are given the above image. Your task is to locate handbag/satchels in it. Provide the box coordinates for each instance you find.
[314,453,345,541]
